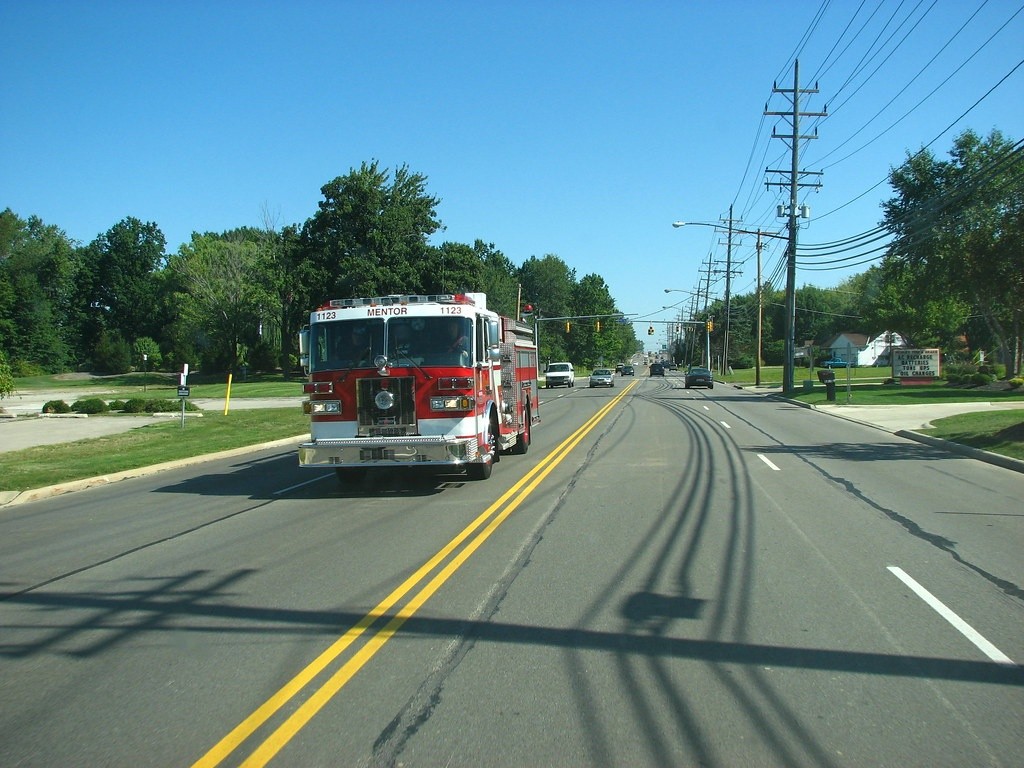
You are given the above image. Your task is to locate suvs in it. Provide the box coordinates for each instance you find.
[649,363,665,377]
[543,363,574,388]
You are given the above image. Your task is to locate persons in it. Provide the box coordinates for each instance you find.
[341,327,378,364]
[442,320,469,359]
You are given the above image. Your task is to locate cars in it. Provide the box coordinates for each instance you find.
[684,368,714,389]
[644,350,678,372]
[621,366,634,377]
[589,370,615,388]
[633,360,638,367]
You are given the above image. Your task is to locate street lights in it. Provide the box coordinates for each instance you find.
[671,221,762,386]
[664,289,726,376]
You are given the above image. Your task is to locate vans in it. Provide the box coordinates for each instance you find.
[615,363,625,373]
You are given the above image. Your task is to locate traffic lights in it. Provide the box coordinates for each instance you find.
[710,322,714,332]
[648,326,655,336]
[676,326,680,333]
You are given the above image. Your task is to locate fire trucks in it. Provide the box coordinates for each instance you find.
[300,291,541,481]
